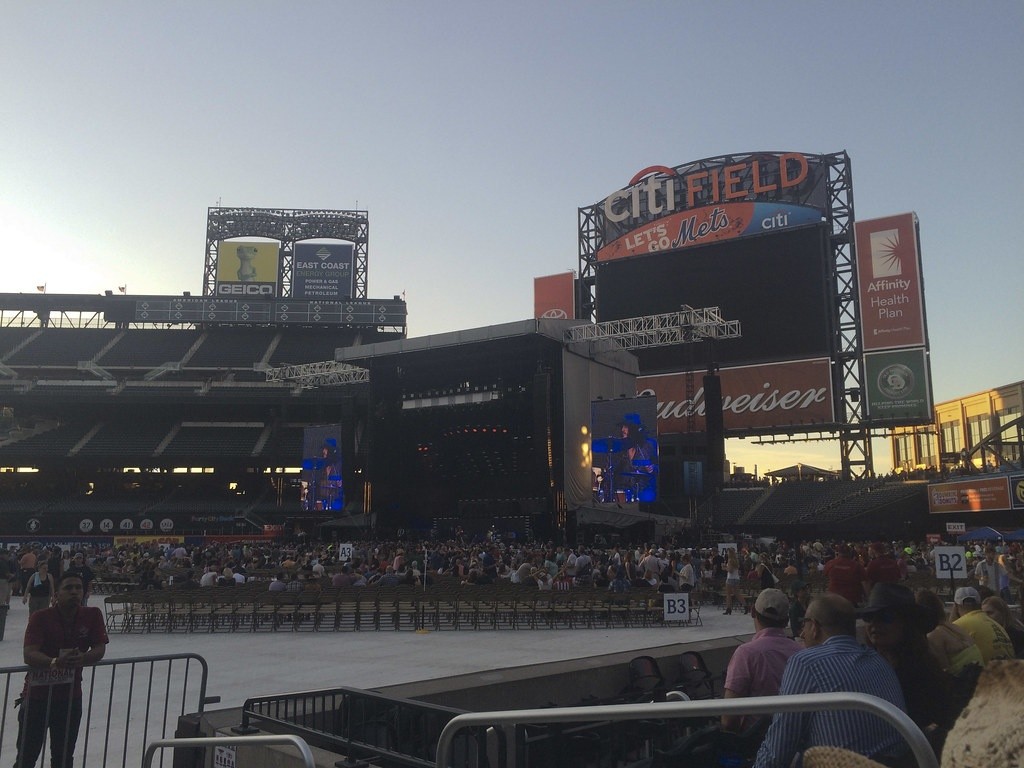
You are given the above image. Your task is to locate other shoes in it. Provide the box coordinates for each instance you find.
[19,593,25,596]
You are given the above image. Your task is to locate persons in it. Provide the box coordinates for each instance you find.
[13,572,110,768]
[322,438,342,494]
[855,581,935,733]
[915,591,986,682]
[749,594,909,768]
[877,460,992,480]
[0,573,11,641]
[606,568,630,602]
[674,554,695,612]
[0,525,1024,604]
[619,413,657,491]
[575,545,593,585]
[981,597,1024,660]
[948,586,1017,669]
[722,548,749,615]
[721,588,807,744]
[22,561,54,618]
[63,553,92,607]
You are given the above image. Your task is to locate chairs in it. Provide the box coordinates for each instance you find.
[93,571,707,631]
[625,651,724,761]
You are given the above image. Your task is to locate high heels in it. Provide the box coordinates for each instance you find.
[744,606,750,614]
[723,607,732,615]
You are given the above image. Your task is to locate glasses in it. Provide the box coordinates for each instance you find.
[985,611,1002,615]
[798,618,822,630]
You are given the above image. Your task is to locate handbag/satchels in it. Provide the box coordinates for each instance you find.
[761,563,779,584]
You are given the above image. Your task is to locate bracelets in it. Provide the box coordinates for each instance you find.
[51,658,56,670]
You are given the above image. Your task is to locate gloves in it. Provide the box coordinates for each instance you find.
[55,645,89,670]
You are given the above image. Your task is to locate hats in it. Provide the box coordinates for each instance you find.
[755,588,789,620]
[904,547,912,555]
[660,549,667,554]
[412,561,417,567]
[868,542,885,553]
[954,587,981,605]
[649,549,655,554]
[74,553,83,559]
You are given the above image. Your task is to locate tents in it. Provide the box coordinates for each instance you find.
[955,526,1004,546]
[1004,528,1024,541]
